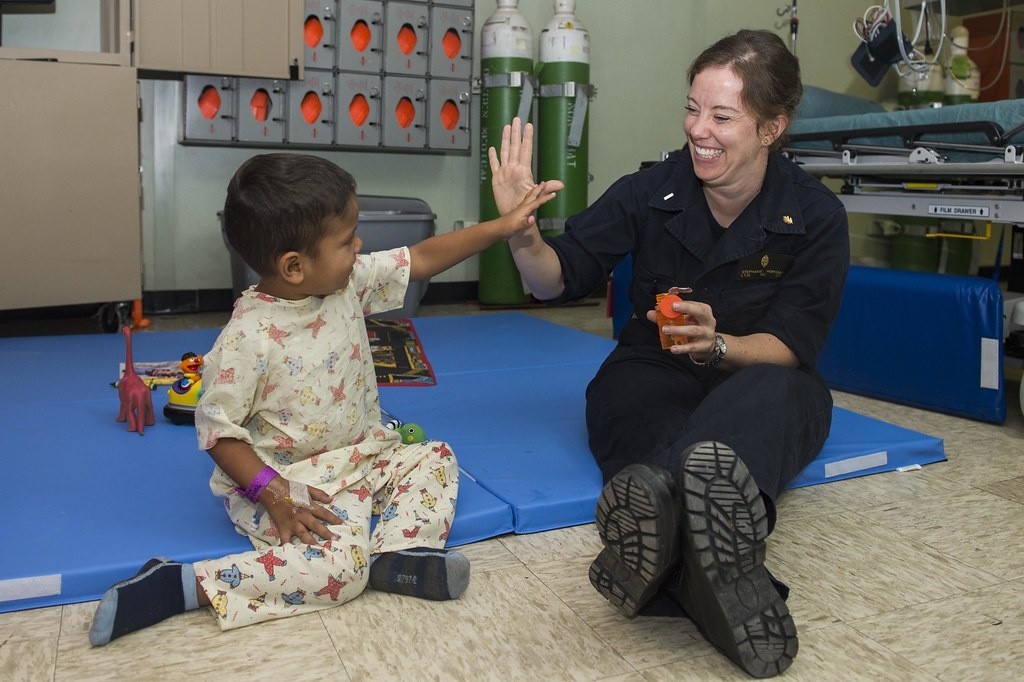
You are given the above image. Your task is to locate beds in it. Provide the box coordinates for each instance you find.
[775,84,1024,229]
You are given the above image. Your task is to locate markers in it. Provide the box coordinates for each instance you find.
[110,366,183,390]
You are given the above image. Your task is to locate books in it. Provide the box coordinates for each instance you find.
[119,362,183,386]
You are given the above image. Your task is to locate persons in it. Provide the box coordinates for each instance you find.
[87,153,555,646]
[488,29,849,679]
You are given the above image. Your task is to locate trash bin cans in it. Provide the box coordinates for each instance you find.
[216,194,440,322]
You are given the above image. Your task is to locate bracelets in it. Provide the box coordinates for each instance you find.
[236,466,280,505]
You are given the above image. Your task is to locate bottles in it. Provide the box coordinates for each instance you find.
[655,294,688,350]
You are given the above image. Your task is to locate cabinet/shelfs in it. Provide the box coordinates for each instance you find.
[0,0,305,312]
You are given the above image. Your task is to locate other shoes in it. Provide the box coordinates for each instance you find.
[665,441,799,679]
[589,464,680,620]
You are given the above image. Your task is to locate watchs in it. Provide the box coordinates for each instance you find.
[689,333,728,366]
